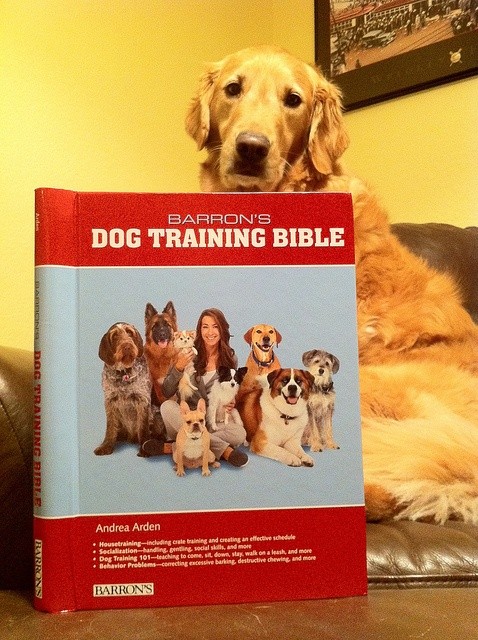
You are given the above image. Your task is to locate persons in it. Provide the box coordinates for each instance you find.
[142,307,248,468]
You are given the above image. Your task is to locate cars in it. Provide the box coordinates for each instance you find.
[360,30,396,50]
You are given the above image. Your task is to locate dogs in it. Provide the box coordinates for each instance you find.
[144,300,180,403]
[206,366,248,432]
[301,348,342,453]
[173,398,221,477]
[239,323,282,443]
[183,44,478,525]
[248,367,315,468]
[93,320,152,457]
[159,329,199,398]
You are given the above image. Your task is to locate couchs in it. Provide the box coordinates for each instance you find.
[0,224,478,587]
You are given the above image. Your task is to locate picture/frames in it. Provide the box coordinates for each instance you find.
[314,0,478,112]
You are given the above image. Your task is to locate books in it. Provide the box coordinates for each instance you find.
[32,186,369,617]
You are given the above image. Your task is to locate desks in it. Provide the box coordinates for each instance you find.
[0,589,478,640]
